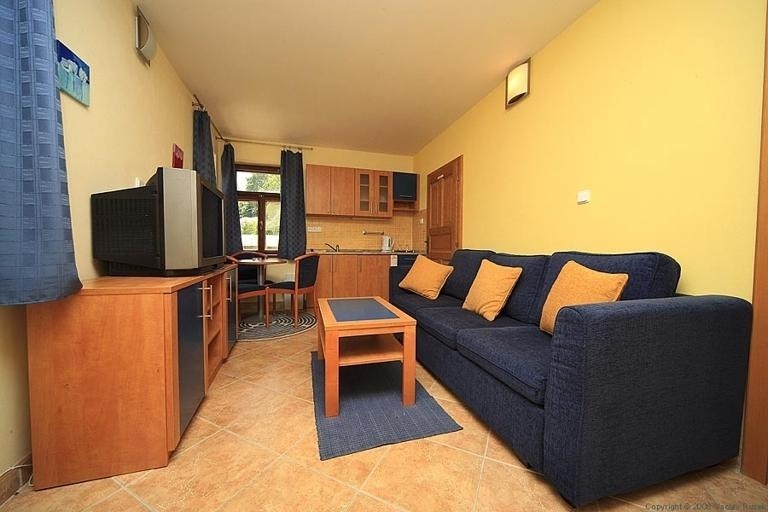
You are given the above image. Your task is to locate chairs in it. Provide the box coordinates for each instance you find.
[269,253,320,326]
[224,254,271,340]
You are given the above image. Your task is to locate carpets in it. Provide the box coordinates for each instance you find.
[311,350,464,462]
[239,309,319,342]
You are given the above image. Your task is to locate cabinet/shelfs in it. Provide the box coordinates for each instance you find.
[306,255,357,310]
[393,171,418,203]
[29,261,239,494]
[305,163,355,220]
[357,254,390,301]
[355,168,393,218]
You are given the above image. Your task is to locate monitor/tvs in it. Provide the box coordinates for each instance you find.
[90,167,226,277]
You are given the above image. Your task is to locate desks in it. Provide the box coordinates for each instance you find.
[241,258,287,320]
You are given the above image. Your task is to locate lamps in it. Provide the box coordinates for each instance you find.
[135,5,158,68]
[505,57,531,110]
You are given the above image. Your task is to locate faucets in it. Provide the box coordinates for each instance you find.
[324,242,339,252]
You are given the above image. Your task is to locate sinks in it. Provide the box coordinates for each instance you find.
[327,248,364,252]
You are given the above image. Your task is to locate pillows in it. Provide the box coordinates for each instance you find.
[463,258,523,323]
[539,260,628,335]
[399,254,454,300]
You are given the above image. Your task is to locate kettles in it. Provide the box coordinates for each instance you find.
[382,234,394,252]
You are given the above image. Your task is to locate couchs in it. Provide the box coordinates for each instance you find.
[389,250,754,510]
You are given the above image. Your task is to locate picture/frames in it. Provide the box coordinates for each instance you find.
[172,143,185,168]
[55,37,91,107]
